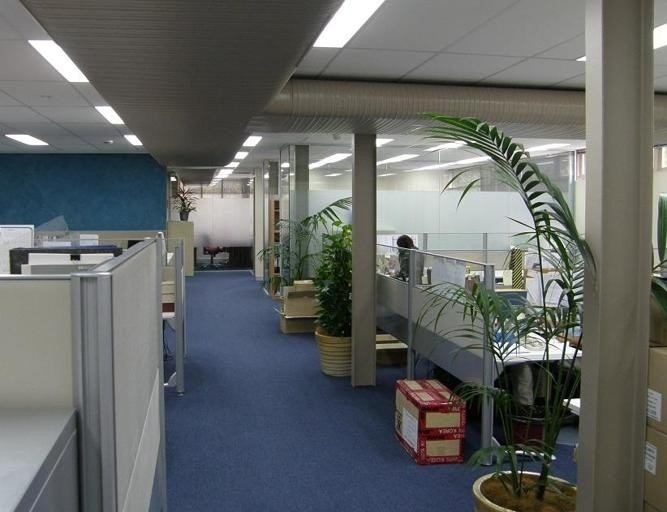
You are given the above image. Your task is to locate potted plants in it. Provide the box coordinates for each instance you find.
[314,222,353,378]
[412,93,577,510]
[171,184,200,222]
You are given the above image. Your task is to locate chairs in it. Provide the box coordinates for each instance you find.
[194,241,231,270]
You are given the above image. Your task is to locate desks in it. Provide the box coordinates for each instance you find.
[364,235,584,406]
[0,224,186,512]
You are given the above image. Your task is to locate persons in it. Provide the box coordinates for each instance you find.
[392,235,425,284]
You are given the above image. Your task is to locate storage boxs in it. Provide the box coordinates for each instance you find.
[644,343,667,512]
[395,377,467,466]
[376,333,408,365]
[278,276,324,335]
